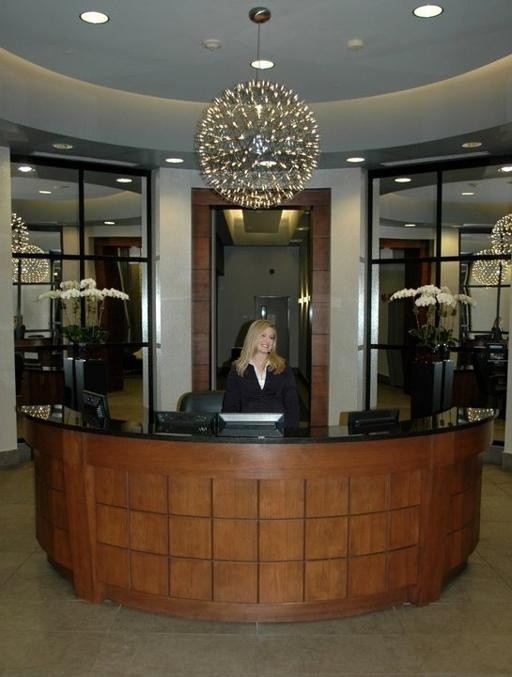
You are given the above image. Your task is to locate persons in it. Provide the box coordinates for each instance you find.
[491,317,503,340]
[223,319,300,427]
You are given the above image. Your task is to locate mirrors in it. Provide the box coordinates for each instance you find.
[363,157,512,445]
[10,154,152,442]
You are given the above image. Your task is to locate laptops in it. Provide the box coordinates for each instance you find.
[23,350,41,366]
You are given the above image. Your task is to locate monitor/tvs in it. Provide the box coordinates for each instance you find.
[79,390,110,418]
[154,411,217,424]
[487,343,506,360]
[216,412,286,425]
[348,408,400,425]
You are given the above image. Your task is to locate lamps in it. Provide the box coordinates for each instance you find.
[190,5,322,211]
